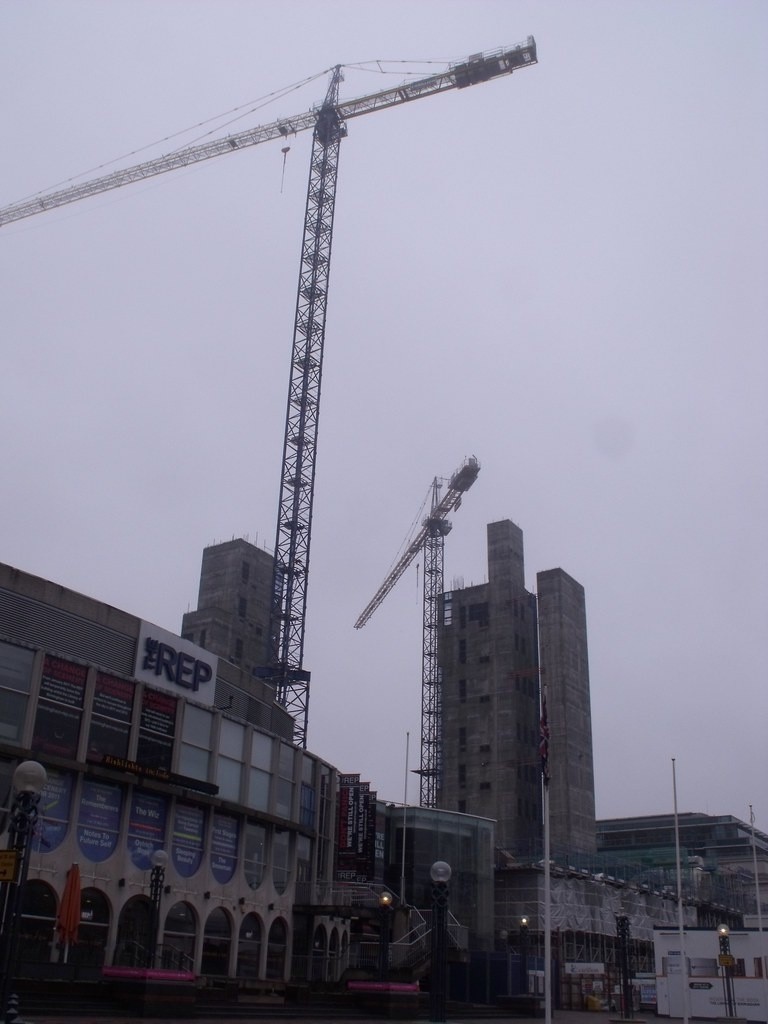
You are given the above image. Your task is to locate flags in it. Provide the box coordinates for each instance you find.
[538,685,552,790]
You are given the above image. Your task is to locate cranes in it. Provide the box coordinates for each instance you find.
[0,36,539,755]
[353,454,482,811]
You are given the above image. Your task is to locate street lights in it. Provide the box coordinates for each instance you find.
[519,918,529,994]
[375,891,392,1010]
[0,760,48,1024]
[147,850,169,968]
[718,928,737,1018]
[429,861,452,1023]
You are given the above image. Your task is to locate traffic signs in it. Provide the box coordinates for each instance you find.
[0,849,18,882]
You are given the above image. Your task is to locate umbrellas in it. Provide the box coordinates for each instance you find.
[53,861,81,965]
